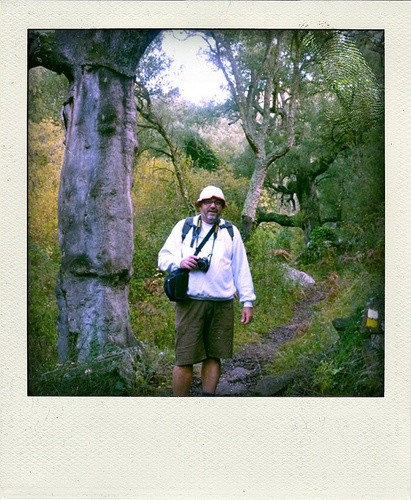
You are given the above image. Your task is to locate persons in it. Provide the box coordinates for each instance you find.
[157,186,258,398]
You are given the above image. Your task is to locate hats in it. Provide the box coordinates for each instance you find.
[196,185,226,206]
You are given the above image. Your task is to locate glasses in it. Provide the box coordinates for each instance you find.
[203,199,222,205]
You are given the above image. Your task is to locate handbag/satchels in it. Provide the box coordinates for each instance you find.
[163,267,190,302]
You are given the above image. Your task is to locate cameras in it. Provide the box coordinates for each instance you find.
[194,257,210,273]
